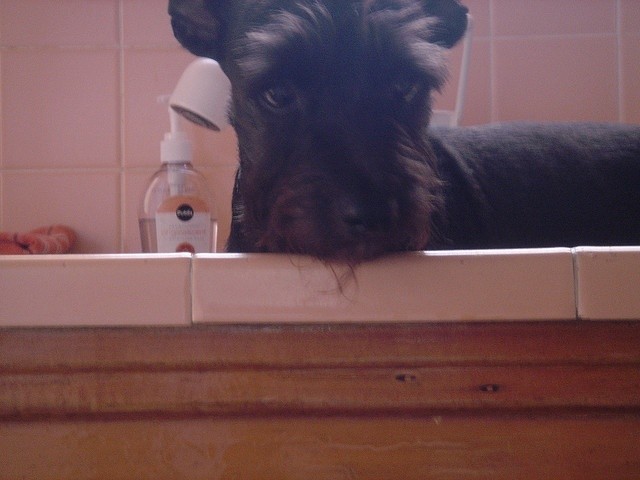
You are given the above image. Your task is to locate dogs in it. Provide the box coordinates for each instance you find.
[168,0,640,302]
